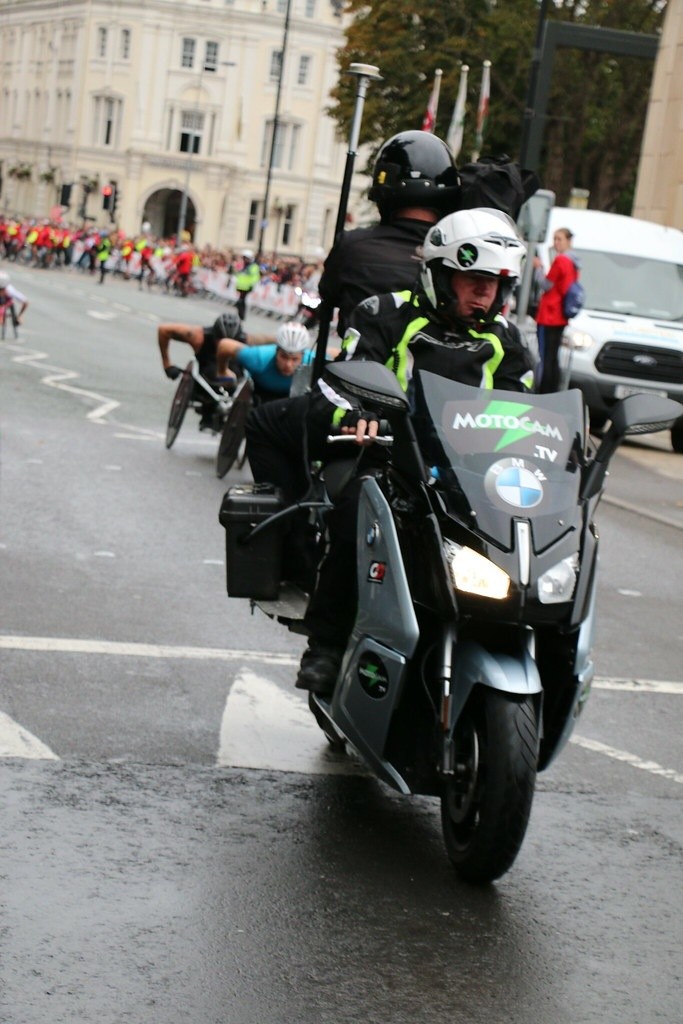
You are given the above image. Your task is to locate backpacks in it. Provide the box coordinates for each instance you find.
[559,280,585,318]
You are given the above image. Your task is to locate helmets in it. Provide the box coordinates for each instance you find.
[214,313,241,339]
[276,322,311,353]
[368,130,461,203]
[415,207,527,334]
[0,273,9,288]
[242,250,255,259]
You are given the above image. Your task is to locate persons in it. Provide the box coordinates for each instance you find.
[216,322,331,406]
[245,130,461,483]
[234,250,260,320]
[296,205,539,692]
[0,214,325,324]
[533,228,581,394]
[157,313,279,401]
[0,271,28,326]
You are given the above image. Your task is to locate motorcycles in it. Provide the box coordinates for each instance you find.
[219,62,683,884]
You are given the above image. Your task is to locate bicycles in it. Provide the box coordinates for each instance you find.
[165,360,249,470]
[0,302,18,339]
[217,378,308,479]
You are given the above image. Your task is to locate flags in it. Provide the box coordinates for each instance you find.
[469,68,490,163]
[422,77,440,134]
[446,72,468,160]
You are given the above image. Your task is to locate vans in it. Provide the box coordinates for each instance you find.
[505,206,683,453]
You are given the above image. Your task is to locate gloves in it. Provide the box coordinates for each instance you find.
[165,365,179,380]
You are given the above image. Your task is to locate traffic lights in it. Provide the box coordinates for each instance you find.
[61,184,71,205]
[102,185,115,210]
